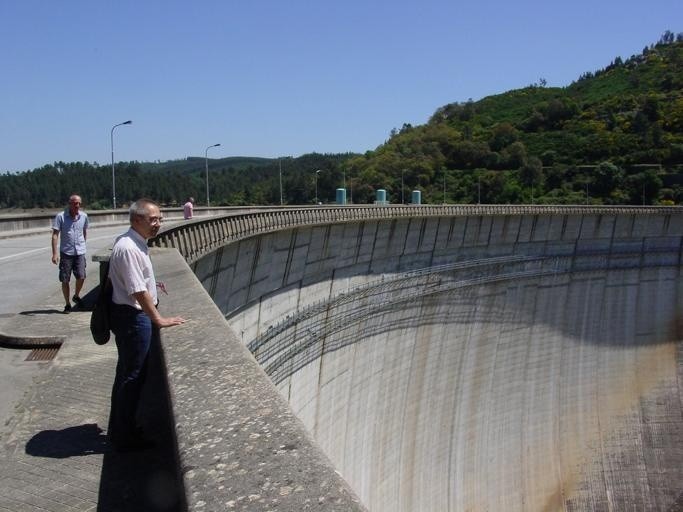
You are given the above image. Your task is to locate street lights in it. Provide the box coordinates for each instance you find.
[205,143,220,206]
[315,169,320,205]
[110,120,132,209]
[278,156,289,205]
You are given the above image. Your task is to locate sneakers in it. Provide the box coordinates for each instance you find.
[63,304,71,313]
[72,296,83,306]
[113,436,153,451]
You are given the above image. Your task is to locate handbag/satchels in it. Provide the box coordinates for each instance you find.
[91,277,113,345]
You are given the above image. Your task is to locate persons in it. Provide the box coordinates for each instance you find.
[104,196,186,456]
[49,194,89,314]
[183,197,194,220]
